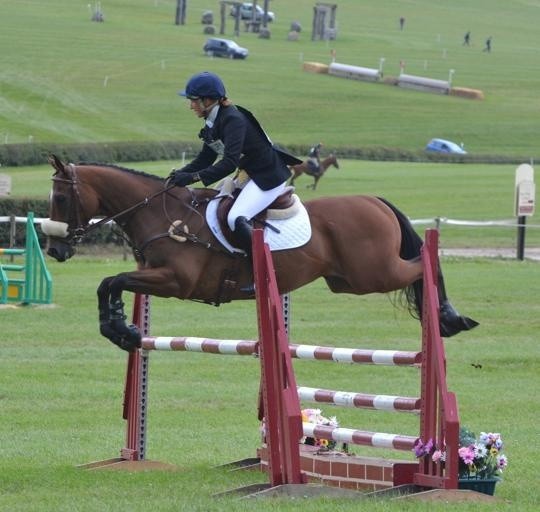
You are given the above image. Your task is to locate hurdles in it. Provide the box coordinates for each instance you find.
[125,230,460,492]
[397,61,456,96]
[0,211,53,304]
[327,49,385,83]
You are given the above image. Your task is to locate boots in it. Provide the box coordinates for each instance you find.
[231,216,255,294]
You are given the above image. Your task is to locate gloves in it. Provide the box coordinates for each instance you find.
[169,164,200,187]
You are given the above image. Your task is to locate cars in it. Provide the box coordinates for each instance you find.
[425,137,469,156]
[231,1,276,22]
[202,37,250,60]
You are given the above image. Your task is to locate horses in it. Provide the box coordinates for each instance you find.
[40,153,481,354]
[286,154,340,192]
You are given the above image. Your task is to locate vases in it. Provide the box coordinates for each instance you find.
[457,480,498,495]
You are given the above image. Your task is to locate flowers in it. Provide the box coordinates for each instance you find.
[258,408,340,449]
[416,430,508,478]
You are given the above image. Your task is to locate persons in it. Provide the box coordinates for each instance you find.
[462,31,471,47]
[169,70,305,297]
[481,36,493,54]
[306,143,324,173]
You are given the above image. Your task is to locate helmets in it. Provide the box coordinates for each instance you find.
[178,72,226,100]
